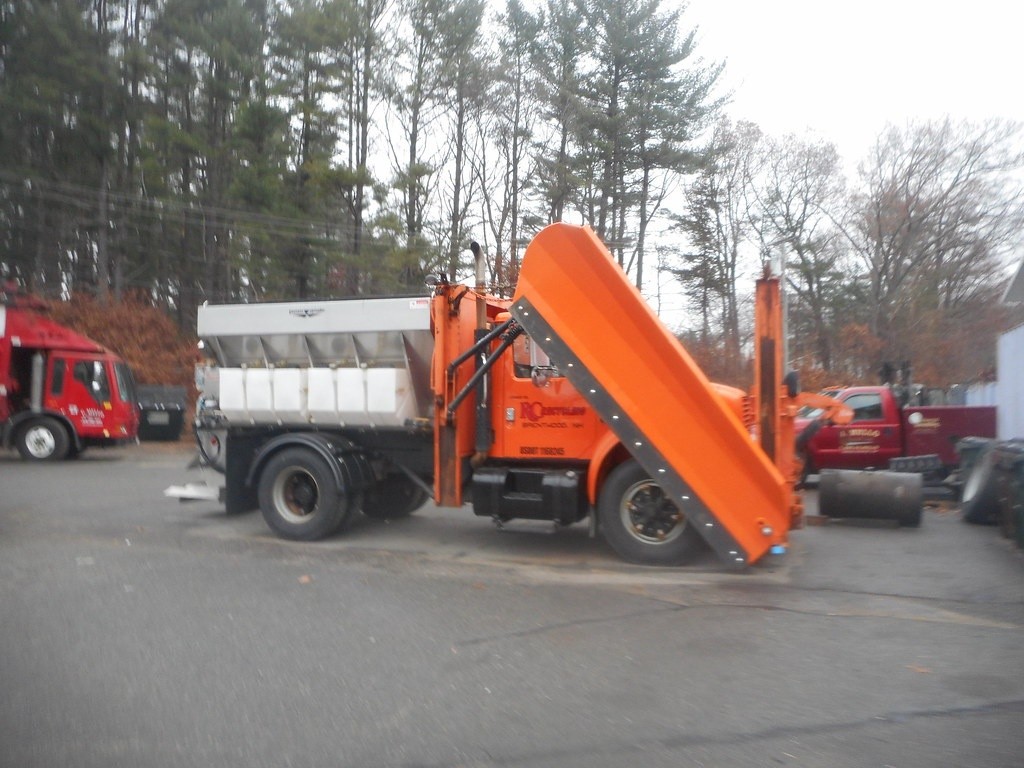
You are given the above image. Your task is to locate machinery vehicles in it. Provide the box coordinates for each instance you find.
[194,222,801,573]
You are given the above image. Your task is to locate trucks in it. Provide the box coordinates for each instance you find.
[790,386,996,483]
[125,300,196,440]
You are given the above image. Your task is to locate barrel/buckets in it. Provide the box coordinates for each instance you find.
[815,467,924,528]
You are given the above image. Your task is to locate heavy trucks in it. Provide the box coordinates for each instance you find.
[2,308,142,461]
